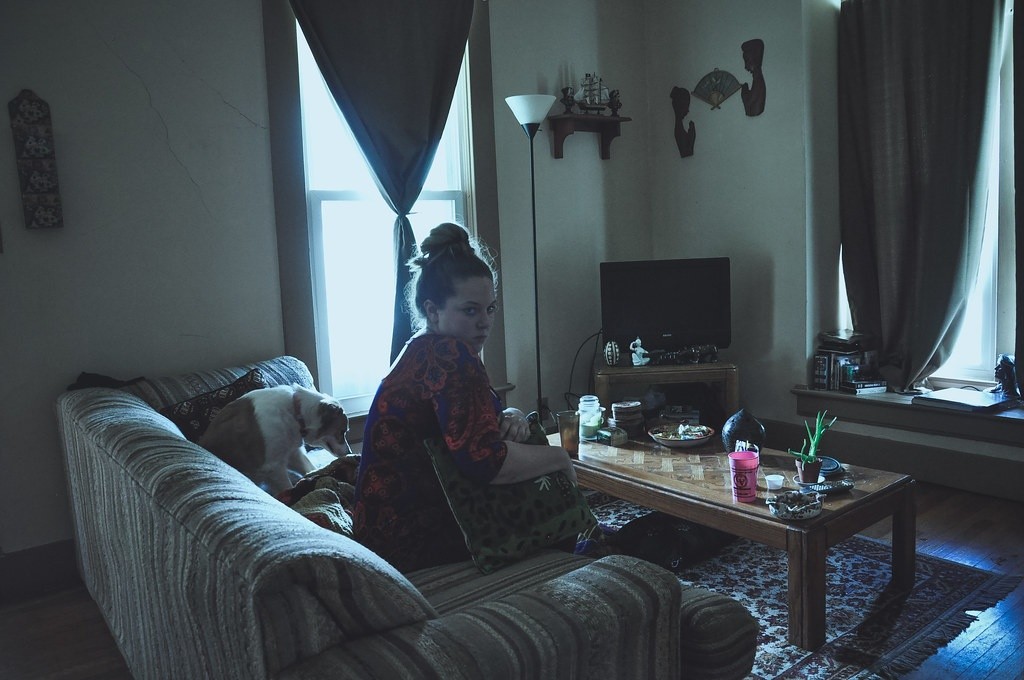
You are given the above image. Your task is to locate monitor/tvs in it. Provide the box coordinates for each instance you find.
[601,257,731,353]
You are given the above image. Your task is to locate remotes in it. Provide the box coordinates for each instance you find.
[800,481,854,496]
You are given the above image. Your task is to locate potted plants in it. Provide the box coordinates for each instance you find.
[788,408,838,482]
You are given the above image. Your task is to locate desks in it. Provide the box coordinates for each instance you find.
[593,352,739,421]
[545,418,918,651]
[792,382,1024,462]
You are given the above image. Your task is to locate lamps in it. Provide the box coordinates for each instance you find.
[504,95,556,433]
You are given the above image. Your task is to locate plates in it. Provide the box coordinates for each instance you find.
[649,425,714,448]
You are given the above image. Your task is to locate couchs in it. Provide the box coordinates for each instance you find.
[57,354,758,680]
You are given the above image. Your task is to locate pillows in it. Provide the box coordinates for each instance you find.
[423,410,599,576]
[158,368,270,444]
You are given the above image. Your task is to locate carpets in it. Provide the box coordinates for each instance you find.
[583,491,1024,680]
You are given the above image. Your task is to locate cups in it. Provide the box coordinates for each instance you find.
[579,396,601,437]
[728,451,758,502]
[765,474,784,490]
[557,411,580,457]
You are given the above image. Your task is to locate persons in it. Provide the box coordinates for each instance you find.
[353,222,597,574]
[994,353,1021,399]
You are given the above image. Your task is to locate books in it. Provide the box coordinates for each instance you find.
[812,328,888,396]
[911,387,1019,415]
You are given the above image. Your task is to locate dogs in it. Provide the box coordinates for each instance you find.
[198,384,353,491]
